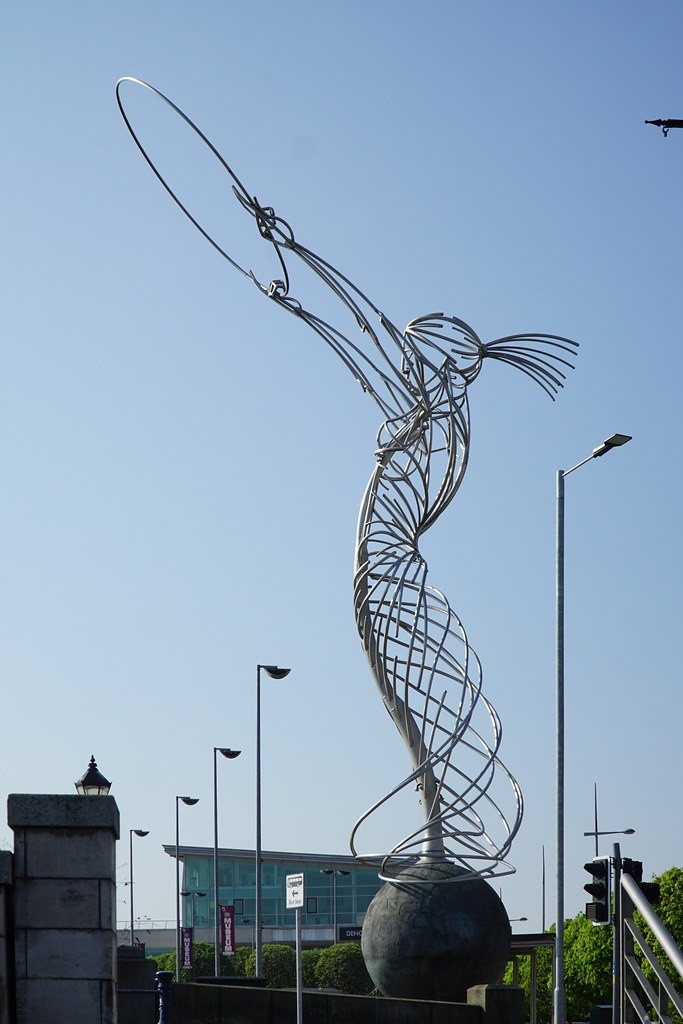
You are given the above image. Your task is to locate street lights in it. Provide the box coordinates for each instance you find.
[129,654,291,983]
[584,782,636,857]
[555,432,633,1023]
[321,868,350,944]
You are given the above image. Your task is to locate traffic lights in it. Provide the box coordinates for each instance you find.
[583,854,664,927]
